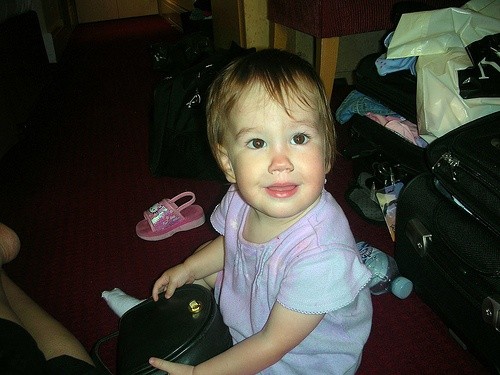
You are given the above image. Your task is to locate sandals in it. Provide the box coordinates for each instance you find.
[136,191,205,241]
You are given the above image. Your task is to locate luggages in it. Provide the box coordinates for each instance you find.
[394,111,500,370]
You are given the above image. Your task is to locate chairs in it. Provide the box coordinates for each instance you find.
[266,0,399,105]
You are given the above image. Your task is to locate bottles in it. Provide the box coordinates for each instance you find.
[355,238,413,299]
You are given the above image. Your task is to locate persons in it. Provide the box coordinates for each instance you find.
[149,48,372,375]
[0,221,101,375]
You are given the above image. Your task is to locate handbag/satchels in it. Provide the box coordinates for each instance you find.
[145,31,231,177]
[91,284,233,375]
